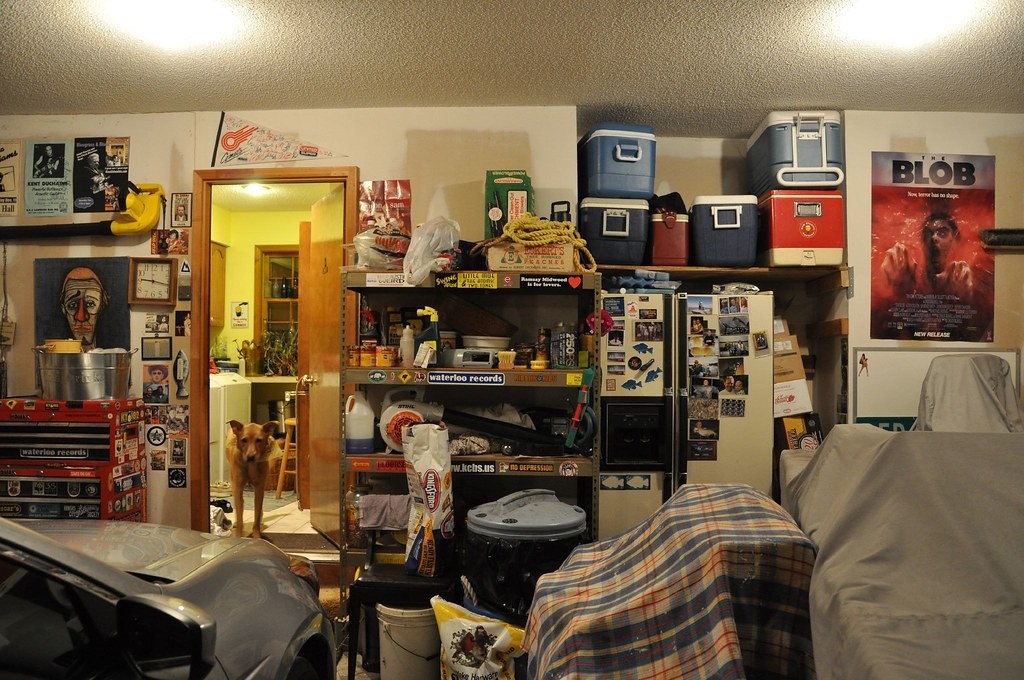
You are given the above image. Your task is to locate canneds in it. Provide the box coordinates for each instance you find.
[345,344,396,366]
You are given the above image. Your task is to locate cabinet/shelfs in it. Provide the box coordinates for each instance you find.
[339,268,602,615]
[210,239,230,326]
[262,250,298,335]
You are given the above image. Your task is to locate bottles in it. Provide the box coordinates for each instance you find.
[272,276,299,298]
[549,201,571,222]
[342,390,374,454]
[346,307,440,367]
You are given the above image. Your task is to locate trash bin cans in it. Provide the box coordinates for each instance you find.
[464,488,589,627]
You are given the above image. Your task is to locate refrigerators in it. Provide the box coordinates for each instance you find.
[598,291,777,543]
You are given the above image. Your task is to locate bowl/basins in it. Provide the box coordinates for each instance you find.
[463,336,511,357]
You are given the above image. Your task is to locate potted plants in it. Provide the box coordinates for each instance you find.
[263,326,300,376]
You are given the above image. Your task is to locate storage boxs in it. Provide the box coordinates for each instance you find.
[484,171,536,239]
[690,195,759,269]
[757,190,843,267]
[651,214,690,266]
[773,379,813,418]
[578,123,656,199]
[782,412,823,450]
[580,197,651,264]
[773,313,789,338]
[486,242,576,273]
[746,111,841,195]
[773,335,806,384]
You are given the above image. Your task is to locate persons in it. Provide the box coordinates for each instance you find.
[721,358,744,375]
[757,335,764,346]
[719,297,748,315]
[718,376,745,395]
[694,380,719,399]
[737,339,749,356]
[691,318,704,335]
[636,322,662,340]
[83,149,120,194]
[692,360,704,377]
[878,212,984,333]
[32,142,62,178]
[703,329,714,346]
[110,206,192,508]
[692,422,711,439]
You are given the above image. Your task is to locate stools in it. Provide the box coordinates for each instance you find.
[347,563,465,680]
[275,418,296,498]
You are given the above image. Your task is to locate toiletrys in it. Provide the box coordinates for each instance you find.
[273,276,298,298]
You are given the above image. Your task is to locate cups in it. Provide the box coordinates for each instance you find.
[440,331,456,350]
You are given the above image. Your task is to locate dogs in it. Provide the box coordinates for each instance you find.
[224,419,280,539]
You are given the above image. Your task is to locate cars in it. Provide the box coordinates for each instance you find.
[0,516,338,680]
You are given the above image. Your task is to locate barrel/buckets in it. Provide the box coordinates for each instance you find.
[31,347,138,399]
[374,600,441,680]
[44,338,84,354]
[269,400,295,442]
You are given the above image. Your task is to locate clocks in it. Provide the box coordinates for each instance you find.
[126,256,177,305]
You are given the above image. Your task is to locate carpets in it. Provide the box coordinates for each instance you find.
[245,531,339,554]
